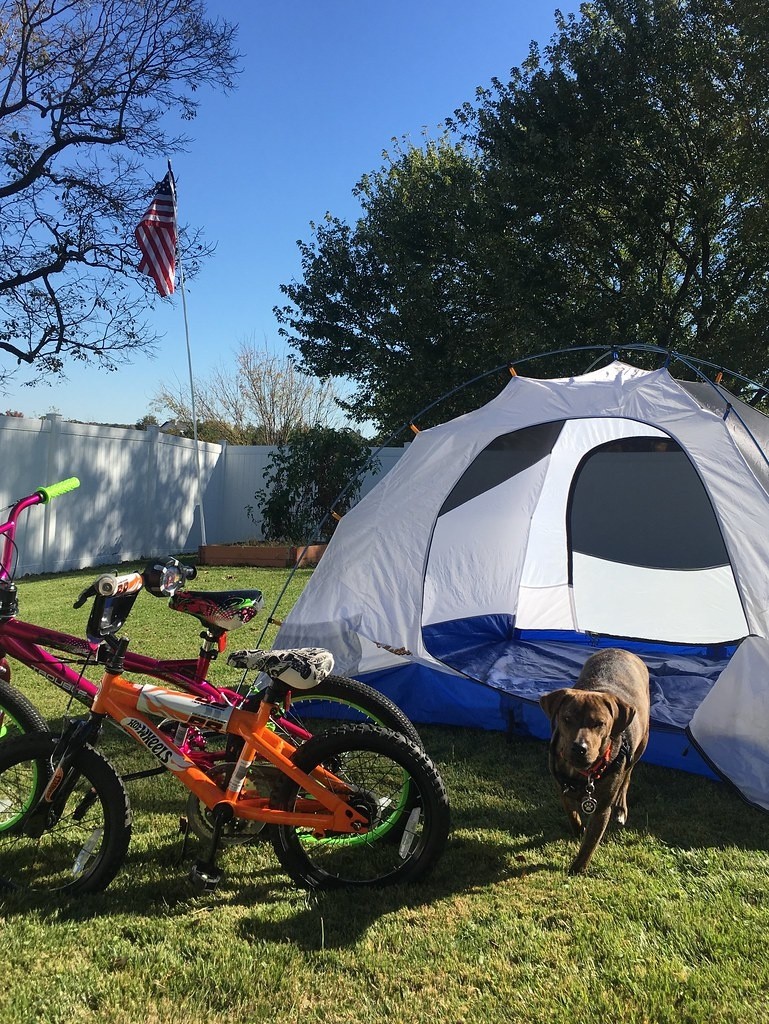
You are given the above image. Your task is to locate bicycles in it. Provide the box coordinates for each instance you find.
[0,556,456,900]
[1,475,426,859]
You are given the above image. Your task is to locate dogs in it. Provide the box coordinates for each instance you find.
[540,647,651,876]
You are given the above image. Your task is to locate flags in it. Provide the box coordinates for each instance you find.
[135,171,178,296]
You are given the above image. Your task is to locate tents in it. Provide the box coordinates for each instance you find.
[238,343,769,810]
